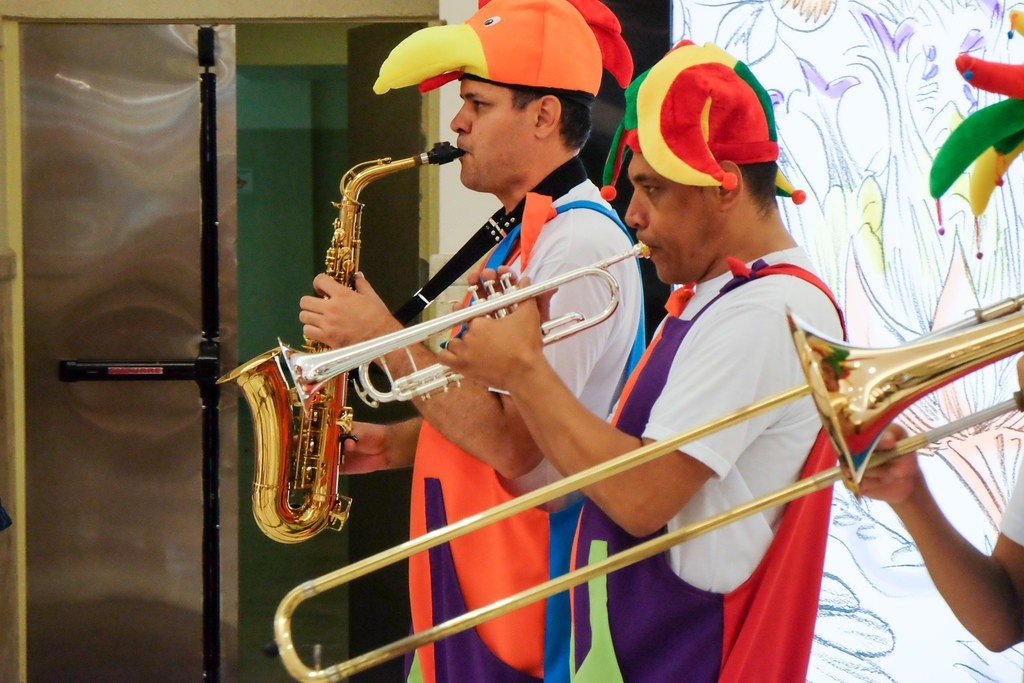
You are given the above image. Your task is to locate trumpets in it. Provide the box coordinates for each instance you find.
[277,239,652,410]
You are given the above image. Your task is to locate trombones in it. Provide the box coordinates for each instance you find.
[271,293,1024,683]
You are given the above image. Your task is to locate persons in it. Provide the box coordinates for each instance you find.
[299,0,643,683]
[433,41,846,683]
[840,353,1024,655]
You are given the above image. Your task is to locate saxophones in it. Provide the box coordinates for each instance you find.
[213,140,466,545]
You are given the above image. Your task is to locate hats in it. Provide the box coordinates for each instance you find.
[371,0,635,97]
[600,41,805,203]
